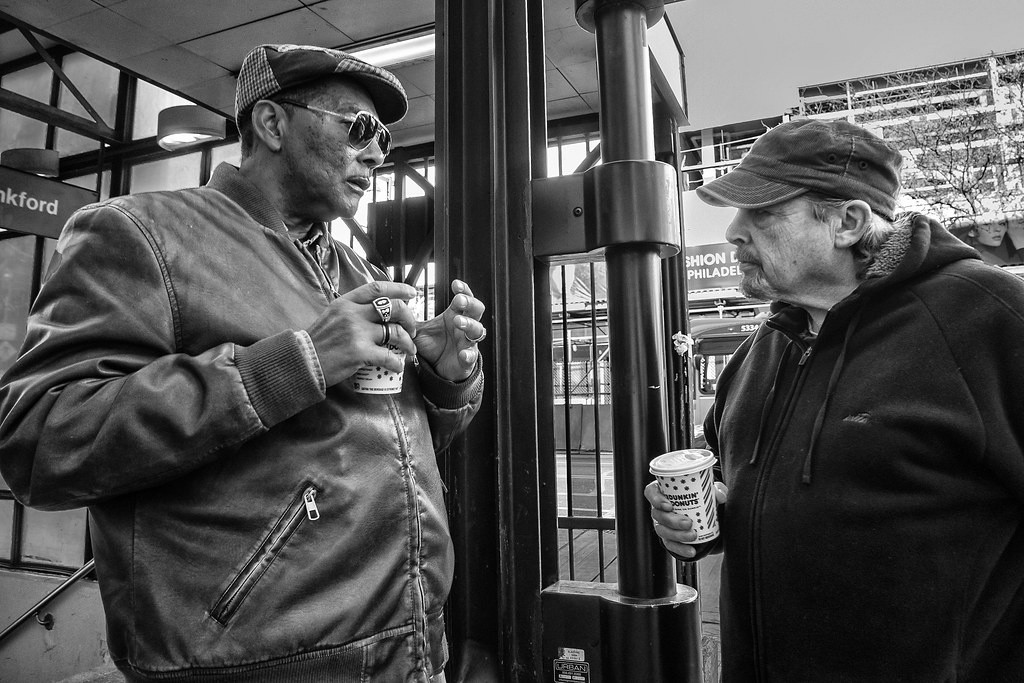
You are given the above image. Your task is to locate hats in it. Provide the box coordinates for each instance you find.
[696,119,904,221]
[235,44,408,138]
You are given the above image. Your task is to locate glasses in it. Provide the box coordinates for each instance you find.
[275,99,392,158]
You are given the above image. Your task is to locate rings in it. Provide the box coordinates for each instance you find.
[380,324,390,346]
[372,296,392,323]
[464,327,486,343]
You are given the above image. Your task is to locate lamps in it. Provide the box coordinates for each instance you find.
[156,105,227,151]
[1,148,59,182]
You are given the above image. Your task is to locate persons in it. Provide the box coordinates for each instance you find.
[968,219,1022,265]
[0,45,486,683]
[648,119,1024,683]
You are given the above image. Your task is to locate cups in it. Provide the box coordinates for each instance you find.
[648,448,720,544]
[342,343,407,394]
